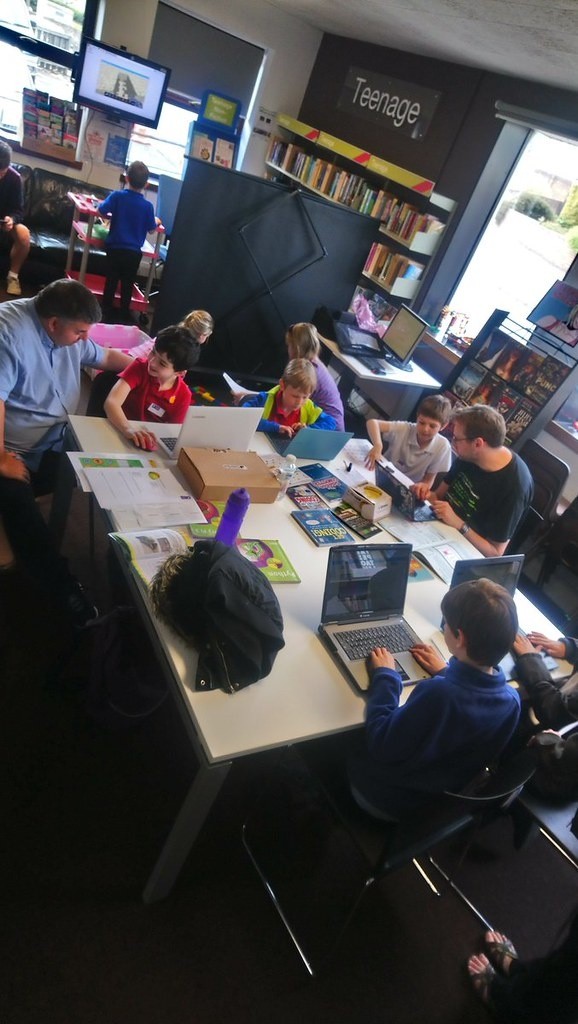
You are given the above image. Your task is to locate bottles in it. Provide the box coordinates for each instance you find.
[273,454,296,500]
[215,487,249,546]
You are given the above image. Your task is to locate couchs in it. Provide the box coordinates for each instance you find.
[13,163,115,287]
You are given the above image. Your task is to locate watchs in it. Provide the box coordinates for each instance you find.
[459,521,471,535]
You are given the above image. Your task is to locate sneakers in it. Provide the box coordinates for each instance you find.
[55,580,99,631]
[6,273,22,296]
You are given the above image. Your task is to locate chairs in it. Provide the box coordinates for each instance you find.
[243,762,538,979]
[505,439,578,590]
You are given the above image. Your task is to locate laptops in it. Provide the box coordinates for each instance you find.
[374,460,439,521]
[318,543,432,693]
[148,406,265,460]
[439,554,559,682]
[265,427,355,462]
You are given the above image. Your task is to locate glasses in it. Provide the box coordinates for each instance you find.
[452,435,486,444]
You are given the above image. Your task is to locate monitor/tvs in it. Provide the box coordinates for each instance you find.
[72,35,172,130]
[382,302,429,372]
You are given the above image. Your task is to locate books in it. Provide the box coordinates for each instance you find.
[285,462,384,548]
[264,137,449,320]
[22,87,78,151]
[108,529,190,596]
[187,500,242,541]
[236,539,301,584]
[375,513,464,584]
[442,330,566,447]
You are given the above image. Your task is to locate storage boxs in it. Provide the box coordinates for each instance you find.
[175,446,281,506]
[342,480,393,523]
[24,86,76,150]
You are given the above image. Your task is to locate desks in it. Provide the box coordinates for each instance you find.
[318,329,441,424]
[67,414,573,906]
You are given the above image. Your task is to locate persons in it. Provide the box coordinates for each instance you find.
[511,627,578,805]
[0,280,134,624]
[241,358,338,439]
[365,394,453,489]
[103,326,201,450]
[231,323,346,432]
[131,310,213,362]
[303,578,520,833]
[0,144,31,295]
[410,405,534,558]
[97,161,162,324]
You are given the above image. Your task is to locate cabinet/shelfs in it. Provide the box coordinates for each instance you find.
[260,114,455,317]
[66,193,166,326]
